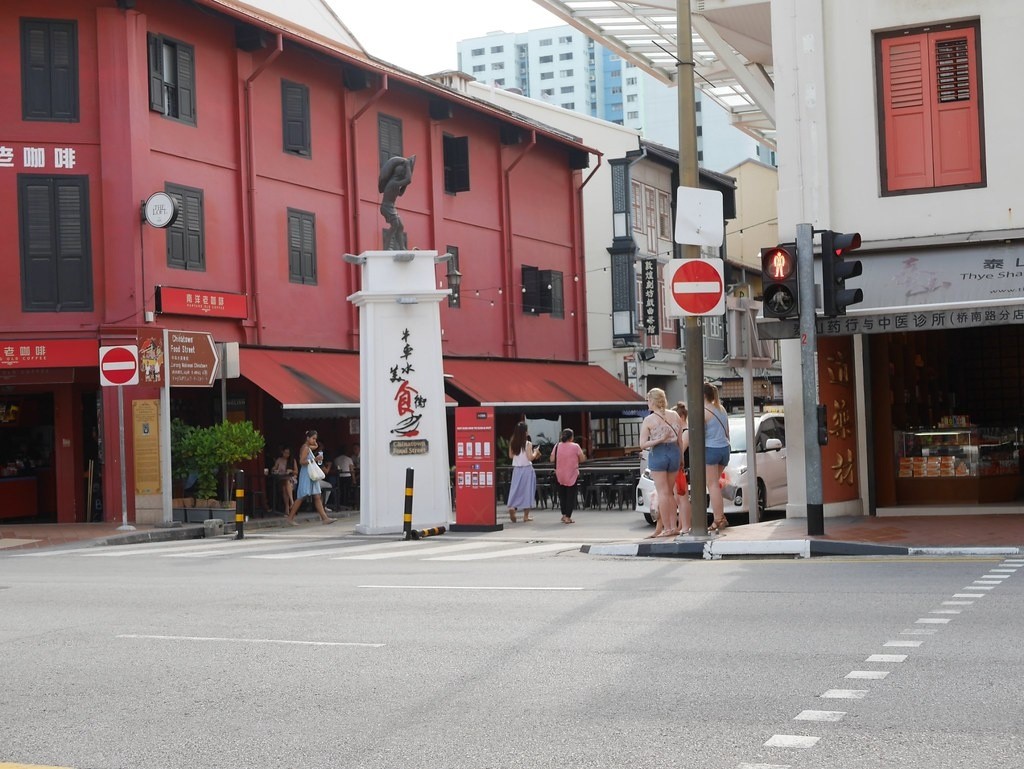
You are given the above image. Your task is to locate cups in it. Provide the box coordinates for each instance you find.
[274,470,279,475]
[264,468,269,476]
[534,449,539,455]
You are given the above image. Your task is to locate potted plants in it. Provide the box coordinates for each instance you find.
[170,417,266,509]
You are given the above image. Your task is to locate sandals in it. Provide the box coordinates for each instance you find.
[669,528,678,535]
[657,530,671,536]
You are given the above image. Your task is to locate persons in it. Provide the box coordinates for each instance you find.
[335,450,356,510]
[639,388,683,537]
[312,440,333,512]
[704,382,731,531]
[507,421,541,524]
[287,429,337,528]
[643,497,663,540]
[670,401,695,533]
[349,444,360,486]
[549,428,586,524]
[272,447,295,518]
[380,159,412,251]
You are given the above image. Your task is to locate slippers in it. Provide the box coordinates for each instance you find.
[565,519,575,524]
[561,517,565,521]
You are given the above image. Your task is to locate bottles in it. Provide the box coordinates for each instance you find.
[318,450,324,465]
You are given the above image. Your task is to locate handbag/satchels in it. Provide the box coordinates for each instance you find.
[719,469,728,488]
[551,472,559,491]
[676,466,686,496]
[307,458,325,482]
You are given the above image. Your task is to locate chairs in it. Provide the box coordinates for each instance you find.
[246,477,265,519]
[321,469,341,512]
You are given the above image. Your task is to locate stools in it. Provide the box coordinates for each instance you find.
[494,469,638,511]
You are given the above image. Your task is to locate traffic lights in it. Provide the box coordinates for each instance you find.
[821,231,864,316]
[817,404,829,446]
[760,245,800,319]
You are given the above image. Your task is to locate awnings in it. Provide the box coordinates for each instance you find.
[229,349,458,420]
[444,360,650,412]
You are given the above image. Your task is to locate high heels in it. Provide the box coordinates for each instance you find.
[722,515,729,526]
[708,520,723,530]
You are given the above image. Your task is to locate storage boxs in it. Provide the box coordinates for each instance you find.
[173,508,186,522]
[186,508,211,522]
[211,508,236,522]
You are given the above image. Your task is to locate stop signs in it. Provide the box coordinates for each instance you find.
[99,345,140,386]
[662,259,726,318]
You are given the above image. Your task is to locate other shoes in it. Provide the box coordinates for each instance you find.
[325,506,332,512]
[289,502,294,510]
[283,511,289,518]
[286,520,299,526]
[509,509,516,522]
[323,518,337,525]
[524,518,533,522]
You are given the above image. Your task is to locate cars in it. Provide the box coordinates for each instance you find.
[636,413,786,528]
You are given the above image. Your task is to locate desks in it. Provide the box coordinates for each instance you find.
[497,456,642,472]
[255,474,289,517]
